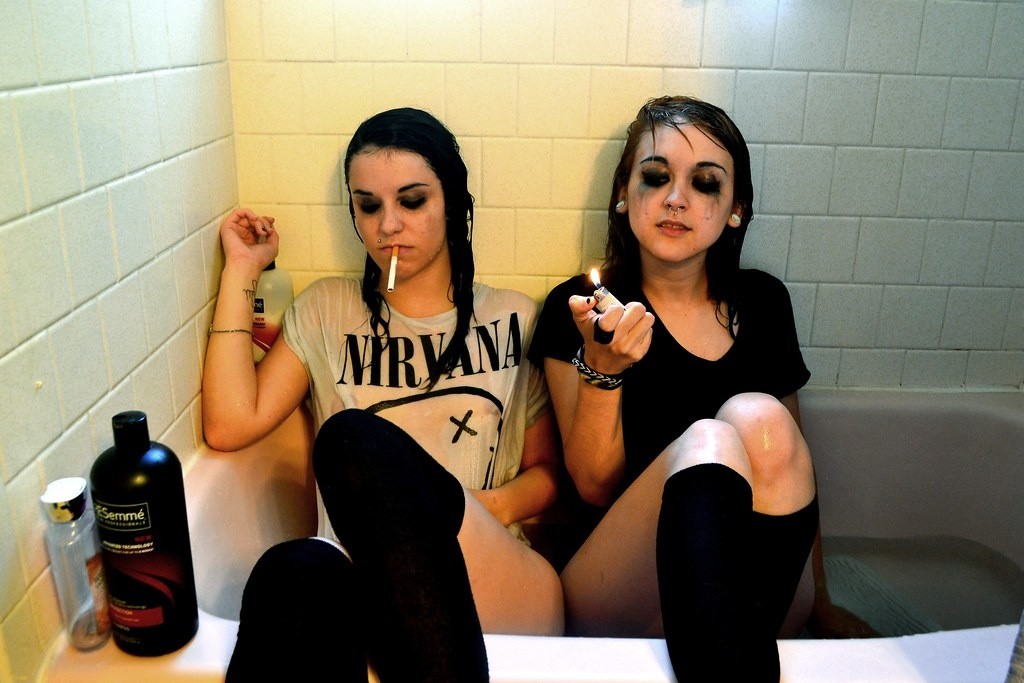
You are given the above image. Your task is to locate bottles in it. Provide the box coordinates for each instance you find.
[88,410,200,658]
[40,477,111,653]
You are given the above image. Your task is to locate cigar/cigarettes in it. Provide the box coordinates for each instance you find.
[387,246,399,292]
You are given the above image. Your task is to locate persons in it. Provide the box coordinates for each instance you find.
[527,96,885,683]
[202,107,565,683]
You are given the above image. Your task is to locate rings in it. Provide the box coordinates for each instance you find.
[593,318,614,344]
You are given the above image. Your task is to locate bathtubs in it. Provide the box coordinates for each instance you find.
[45,387,1024,683]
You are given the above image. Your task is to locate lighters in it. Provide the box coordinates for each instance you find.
[589,268,624,313]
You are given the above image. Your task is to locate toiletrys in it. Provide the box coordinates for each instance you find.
[249,257,297,364]
[37,409,202,661]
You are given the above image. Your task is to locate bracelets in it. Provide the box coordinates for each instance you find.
[208,325,254,336]
[572,346,625,390]
